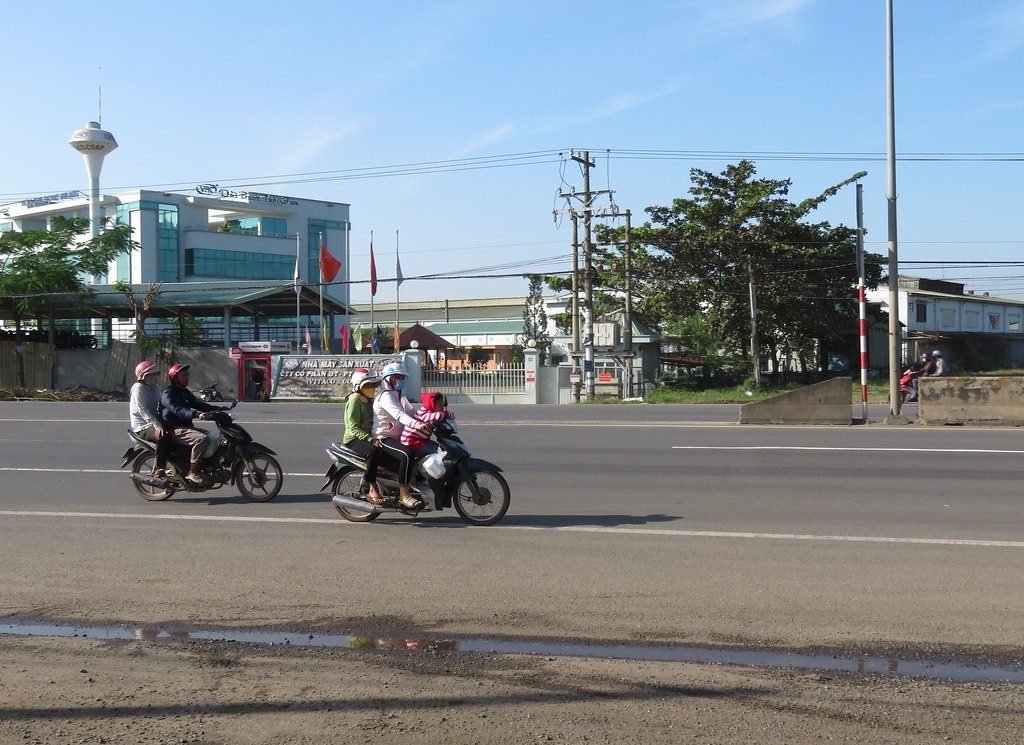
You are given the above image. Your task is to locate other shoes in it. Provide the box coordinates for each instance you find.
[159,468,174,477]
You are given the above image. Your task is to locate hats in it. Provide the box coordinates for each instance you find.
[359,378,381,389]
[139,367,160,381]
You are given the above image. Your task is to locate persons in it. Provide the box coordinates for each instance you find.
[158,364,229,485]
[908,353,934,400]
[371,364,430,507]
[341,368,386,505]
[400,391,455,489]
[130,361,170,479]
[930,350,947,377]
[254,366,262,399]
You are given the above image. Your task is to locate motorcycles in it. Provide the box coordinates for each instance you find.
[199,382,224,402]
[888,365,921,402]
[319,395,511,526]
[120,399,284,503]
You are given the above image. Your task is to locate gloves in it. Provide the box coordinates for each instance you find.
[444,411,455,420]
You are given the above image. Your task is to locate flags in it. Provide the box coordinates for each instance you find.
[305,327,313,356]
[351,322,363,351]
[323,320,330,352]
[392,321,399,350]
[373,324,383,350]
[293,258,302,293]
[396,257,404,287]
[338,322,347,351]
[370,243,377,295]
[318,240,341,283]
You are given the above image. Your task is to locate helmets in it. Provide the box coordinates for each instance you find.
[351,369,376,392]
[921,353,929,360]
[169,364,190,383]
[135,361,157,378]
[932,350,941,357]
[381,363,409,381]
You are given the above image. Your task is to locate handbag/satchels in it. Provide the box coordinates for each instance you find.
[423,446,448,480]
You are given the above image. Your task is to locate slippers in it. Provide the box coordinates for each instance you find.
[366,494,387,504]
[398,495,422,508]
[185,472,204,484]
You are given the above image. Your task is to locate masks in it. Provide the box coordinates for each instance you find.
[360,388,374,398]
[144,376,159,386]
[394,378,406,390]
[923,358,927,362]
